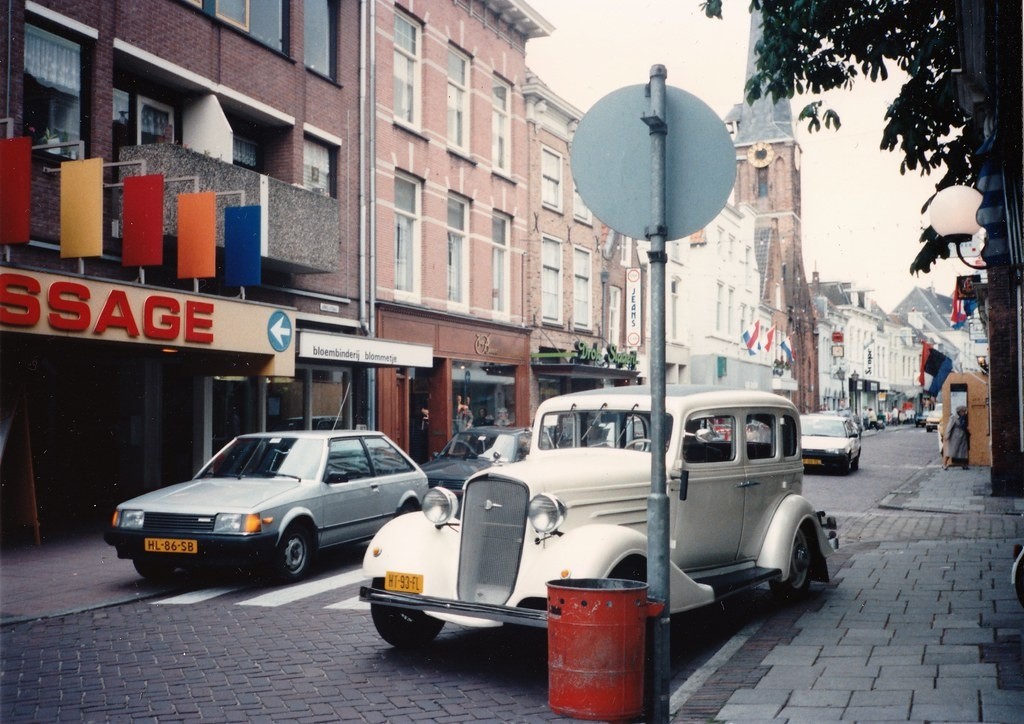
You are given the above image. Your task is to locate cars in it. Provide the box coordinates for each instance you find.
[797,413,863,473]
[414,419,573,497]
[103,428,428,584]
[925,411,942,431]
[357,380,841,672]
[915,413,927,426]
[541,413,724,450]
[254,418,346,432]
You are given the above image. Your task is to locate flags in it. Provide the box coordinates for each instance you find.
[920,343,953,398]
[743,320,762,356]
[764,328,774,352]
[779,336,796,363]
[950,288,978,331]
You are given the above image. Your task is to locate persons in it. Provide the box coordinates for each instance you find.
[839,399,848,410]
[941,405,970,470]
[891,406,899,426]
[457,396,473,431]
[863,406,880,432]
[877,409,886,423]
[475,408,493,426]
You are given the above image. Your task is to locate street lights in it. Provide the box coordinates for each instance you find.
[837,368,845,399]
[850,371,859,412]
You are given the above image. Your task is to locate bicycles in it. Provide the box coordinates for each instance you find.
[1009,543,1024,610]
